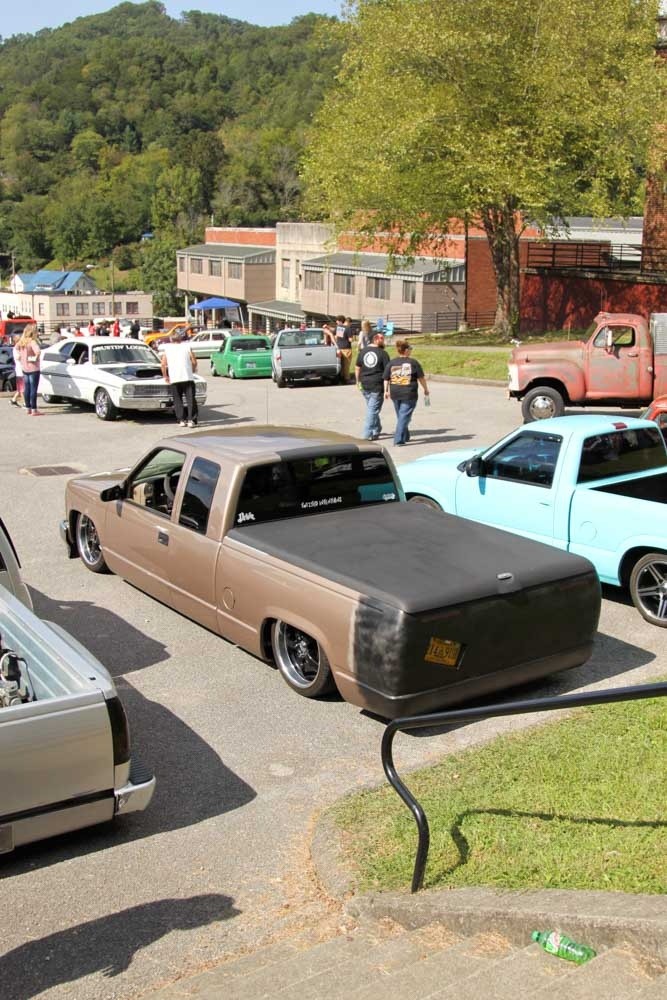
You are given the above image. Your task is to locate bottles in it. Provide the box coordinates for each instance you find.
[424,395,431,408]
[531,930,596,966]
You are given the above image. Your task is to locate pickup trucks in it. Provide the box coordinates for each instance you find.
[507,312,667,424]
[392,413,667,628]
[0,518,160,856]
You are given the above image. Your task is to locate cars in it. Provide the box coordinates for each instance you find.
[57,424,604,723]
[158,328,242,358]
[0,317,206,392]
[209,335,273,379]
[37,335,207,421]
[271,327,341,389]
[639,394,667,439]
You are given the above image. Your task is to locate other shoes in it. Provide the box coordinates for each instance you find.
[26,408,32,414]
[187,420,199,428]
[375,426,382,432]
[179,420,187,426]
[405,431,410,441]
[32,409,44,416]
[368,435,378,441]
[9,400,21,408]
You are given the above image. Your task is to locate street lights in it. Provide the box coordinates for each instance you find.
[86,260,116,317]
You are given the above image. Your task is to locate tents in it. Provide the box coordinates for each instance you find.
[188,298,248,334]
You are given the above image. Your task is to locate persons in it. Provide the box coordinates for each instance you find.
[7,310,201,427]
[265,316,429,447]
